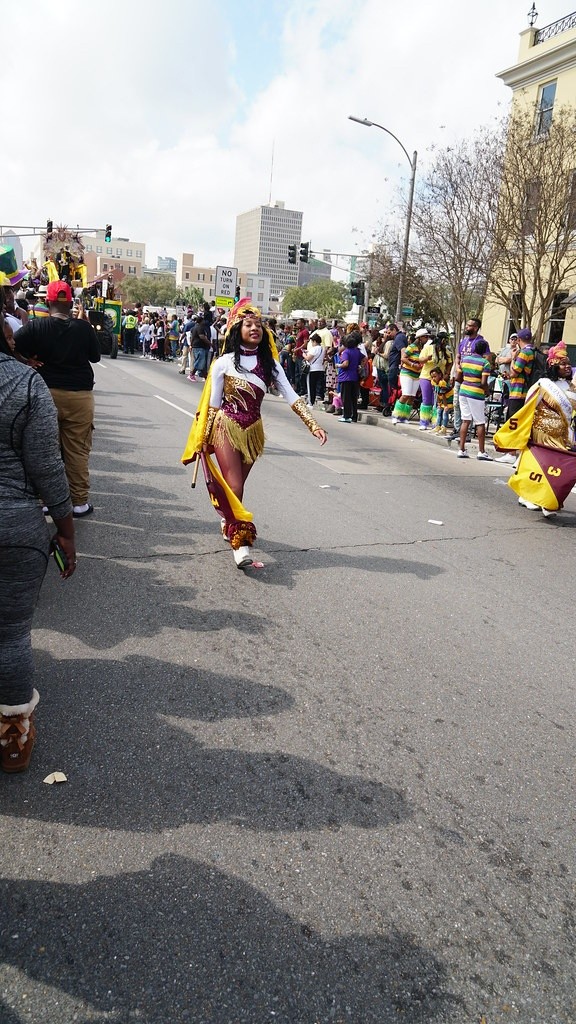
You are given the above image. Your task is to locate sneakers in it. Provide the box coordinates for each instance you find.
[457,448,517,466]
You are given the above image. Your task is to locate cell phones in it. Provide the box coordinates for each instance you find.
[499,372,507,378]
[75,298,80,306]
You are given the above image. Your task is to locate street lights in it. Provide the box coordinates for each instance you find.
[348,114,418,324]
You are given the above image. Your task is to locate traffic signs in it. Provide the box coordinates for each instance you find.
[215,296,234,307]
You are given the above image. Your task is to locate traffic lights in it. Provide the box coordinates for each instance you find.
[234,285,240,302]
[300,241,309,263]
[351,280,364,305]
[288,244,296,264]
[46,220,52,240]
[105,224,112,242]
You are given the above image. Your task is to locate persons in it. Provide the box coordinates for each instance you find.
[388,324,409,402]
[4,322,15,351]
[391,328,428,425]
[335,338,368,423]
[429,368,454,437]
[1,308,78,768]
[494,329,534,469]
[538,344,550,353]
[495,333,520,423]
[0,299,392,410]
[458,341,495,461]
[56,248,72,279]
[303,334,325,409]
[13,282,101,517]
[193,299,328,567]
[486,353,497,364]
[518,341,576,517]
[420,331,452,430]
[444,318,491,443]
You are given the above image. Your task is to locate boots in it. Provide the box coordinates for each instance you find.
[390,399,438,430]
[0,688,39,772]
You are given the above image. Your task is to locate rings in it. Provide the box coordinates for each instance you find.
[74,560,78,565]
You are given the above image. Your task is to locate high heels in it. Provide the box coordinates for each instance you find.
[179,370,185,374]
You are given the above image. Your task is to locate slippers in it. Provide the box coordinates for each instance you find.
[43,510,49,515]
[73,503,93,517]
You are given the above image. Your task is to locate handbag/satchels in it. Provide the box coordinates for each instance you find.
[301,360,311,374]
[139,337,144,342]
[358,361,368,380]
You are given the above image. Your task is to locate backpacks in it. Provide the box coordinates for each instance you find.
[522,347,548,387]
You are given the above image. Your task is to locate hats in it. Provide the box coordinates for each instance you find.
[310,334,321,344]
[517,329,532,339]
[414,328,431,338]
[46,280,72,302]
[360,322,369,328]
[510,333,518,338]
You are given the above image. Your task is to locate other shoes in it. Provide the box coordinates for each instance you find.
[428,425,471,442]
[518,495,558,519]
[187,375,197,382]
[233,545,252,567]
[220,518,230,540]
[322,400,368,423]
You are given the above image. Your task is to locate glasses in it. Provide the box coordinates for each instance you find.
[511,338,515,340]
[363,326,368,329]
